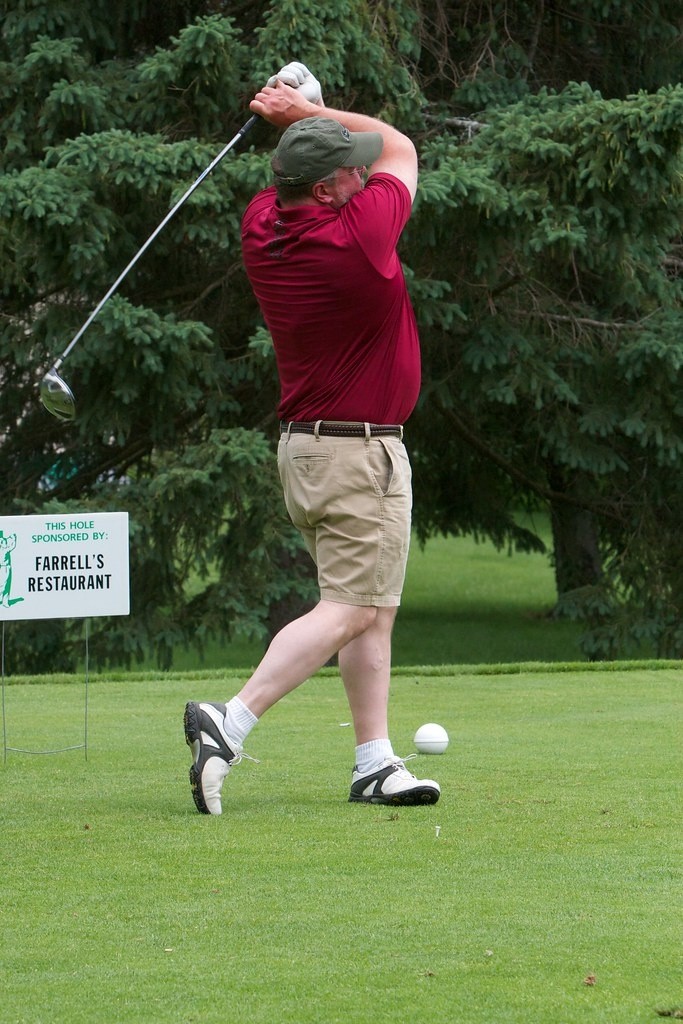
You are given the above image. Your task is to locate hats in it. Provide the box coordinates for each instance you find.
[272,116,384,186]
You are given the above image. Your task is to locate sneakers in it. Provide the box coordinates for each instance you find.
[348,753,441,805]
[183,701,259,816]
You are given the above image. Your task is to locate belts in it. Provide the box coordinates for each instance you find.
[280,421,401,438]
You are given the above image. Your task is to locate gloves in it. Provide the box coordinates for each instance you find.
[266,62,321,104]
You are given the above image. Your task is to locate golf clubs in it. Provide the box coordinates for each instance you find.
[41,114,257,422]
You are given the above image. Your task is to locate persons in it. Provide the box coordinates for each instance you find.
[184,61,442,815]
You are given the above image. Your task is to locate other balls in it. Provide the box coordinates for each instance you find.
[414,723,448,755]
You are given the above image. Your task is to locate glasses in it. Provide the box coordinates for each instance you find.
[328,166,363,179]
